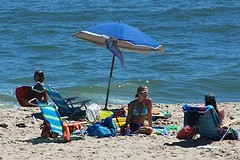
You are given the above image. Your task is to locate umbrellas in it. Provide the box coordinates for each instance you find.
[72,20,164,110]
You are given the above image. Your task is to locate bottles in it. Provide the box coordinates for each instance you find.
[125,124,130,137]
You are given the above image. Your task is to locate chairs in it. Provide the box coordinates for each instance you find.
[43,84,92,110]
[28,97,86,141]
[182,103,238,141]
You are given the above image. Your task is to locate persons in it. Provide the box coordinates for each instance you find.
[117,86,153,135]
[24,70,46,104]
[204,93,224,127]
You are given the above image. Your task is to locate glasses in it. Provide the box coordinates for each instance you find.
[141,91,149,95]
